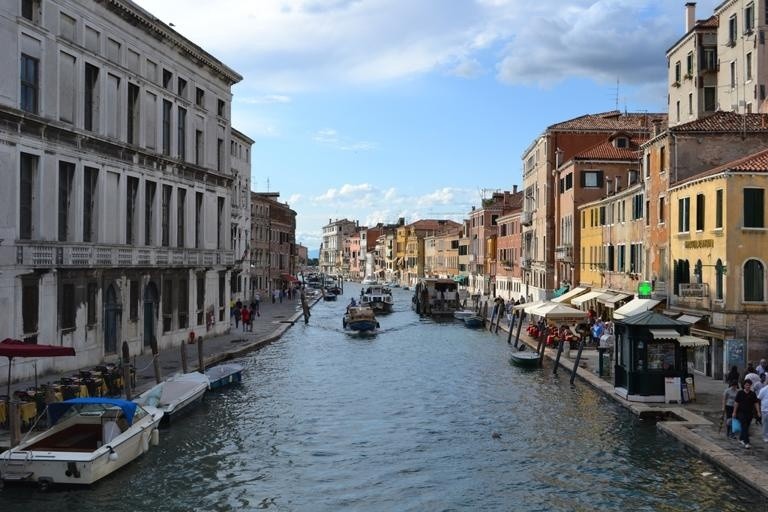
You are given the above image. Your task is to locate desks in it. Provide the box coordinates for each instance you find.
[528,320,579,348]
[0,362,136,434]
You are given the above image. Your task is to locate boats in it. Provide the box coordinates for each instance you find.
[133,371,209,427]
[205,362,246,391]
[510,350,540,368]
[305,271,486,335]
[0,397,163,488]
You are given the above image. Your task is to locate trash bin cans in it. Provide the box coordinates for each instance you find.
[598,347,611,377]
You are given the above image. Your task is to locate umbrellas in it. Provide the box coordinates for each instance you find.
[0,338,75,402]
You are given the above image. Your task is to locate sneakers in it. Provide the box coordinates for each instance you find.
[762,438,767,442]
[729,436,752,449]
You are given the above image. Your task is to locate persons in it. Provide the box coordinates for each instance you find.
[366,286,392,295]
[477,288,613,353]
[230,283,311,331]
[346,297,356,313]
[361,287,365,295]
[719,359,768,448]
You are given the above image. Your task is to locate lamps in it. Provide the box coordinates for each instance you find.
[693,265,728,277]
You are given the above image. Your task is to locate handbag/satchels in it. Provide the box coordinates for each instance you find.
[732,419,741,433]
